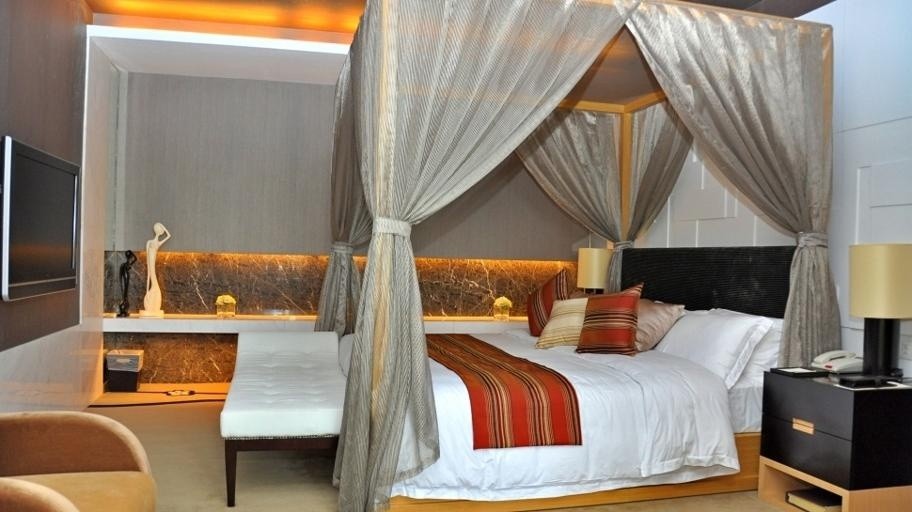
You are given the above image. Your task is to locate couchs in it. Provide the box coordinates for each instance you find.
[3,413,161,511]
[217,333,349,508]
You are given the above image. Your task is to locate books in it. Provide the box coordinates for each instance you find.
[785,486,842,512]
[839,376,898,389]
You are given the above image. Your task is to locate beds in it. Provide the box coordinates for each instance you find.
[342,244,801,509]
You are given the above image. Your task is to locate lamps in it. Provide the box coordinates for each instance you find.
[839,244,912,387]
[574,248,609,296]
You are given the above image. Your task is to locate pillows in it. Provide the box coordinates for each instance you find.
[636,298,686,350]
[535,299,590,347]
[523,267,572,337]
[656,308,773,392]
[576,283,644,359]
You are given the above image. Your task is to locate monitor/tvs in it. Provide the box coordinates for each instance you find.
[0,134,81,303]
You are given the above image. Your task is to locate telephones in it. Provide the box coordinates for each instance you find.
[810,350,863,375]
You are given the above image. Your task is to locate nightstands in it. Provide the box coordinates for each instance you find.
[756,361,912,512]
